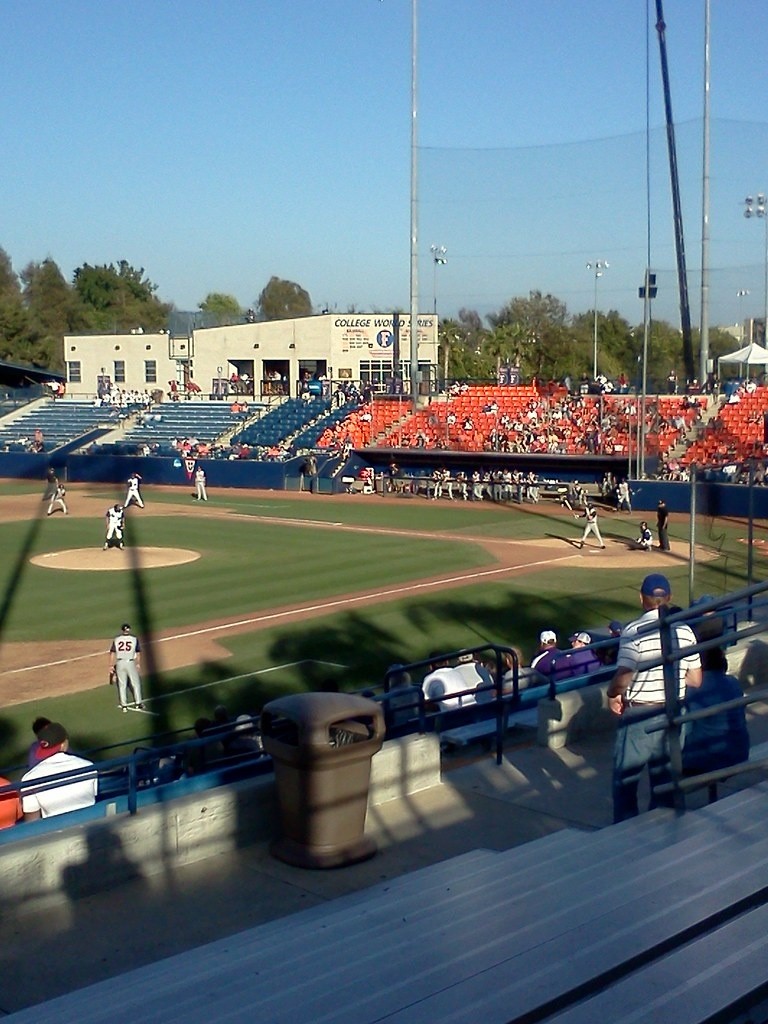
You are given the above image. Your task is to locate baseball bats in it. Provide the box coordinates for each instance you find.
[564,499,577,518]
[635,488,643,494]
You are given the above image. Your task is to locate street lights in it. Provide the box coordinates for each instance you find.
[586,260,610,380]
[430,244,448,313]
[736,290,751,377]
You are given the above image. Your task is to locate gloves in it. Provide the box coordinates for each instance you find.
[586,507,589,515]
[574,515,579,518]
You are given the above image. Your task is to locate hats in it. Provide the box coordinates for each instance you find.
[568,632,591,643]
[608,621,622,632]
[540,631,556,644]
[640,522,647,526]
[235,715,252,730]
[458,649,472,662]
[35,723,67,758]
[122,624,130,631]
[641,573,670,596]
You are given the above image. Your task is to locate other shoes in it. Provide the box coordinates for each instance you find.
[120,543,125,550]
[103,543,108,550]
[136,704,146,709]
[123,707,128,712]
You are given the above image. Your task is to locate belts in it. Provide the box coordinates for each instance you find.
[625,702,665,704]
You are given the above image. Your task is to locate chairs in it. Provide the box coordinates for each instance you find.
[0,387,768,483]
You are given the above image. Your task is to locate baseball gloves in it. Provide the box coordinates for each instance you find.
[110,670,117,685]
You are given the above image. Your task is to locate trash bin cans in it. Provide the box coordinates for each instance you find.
[260,692,387,870]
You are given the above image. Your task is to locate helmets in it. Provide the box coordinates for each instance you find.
[588,503,592,507]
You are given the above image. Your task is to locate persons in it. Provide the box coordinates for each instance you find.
[656,499,671,552]
[0,593,725,833]
[2,430,45,453]
[635,522,654,551]
[123,472,145,508]
[43,378,65,402]
[91,370,768,486]
[104,504,126,550]
[108,622,145,712]
[609,574,702,824]
[575,503,605,549]
[381,462,635,516]
[194,466,208,501]
[43,467,59,501]
[680,646,751,775]
[48,484,68,516]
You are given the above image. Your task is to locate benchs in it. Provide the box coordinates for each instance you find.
[0,788,768,1024]
[539,490,568,500]
[570,483,603,496]
